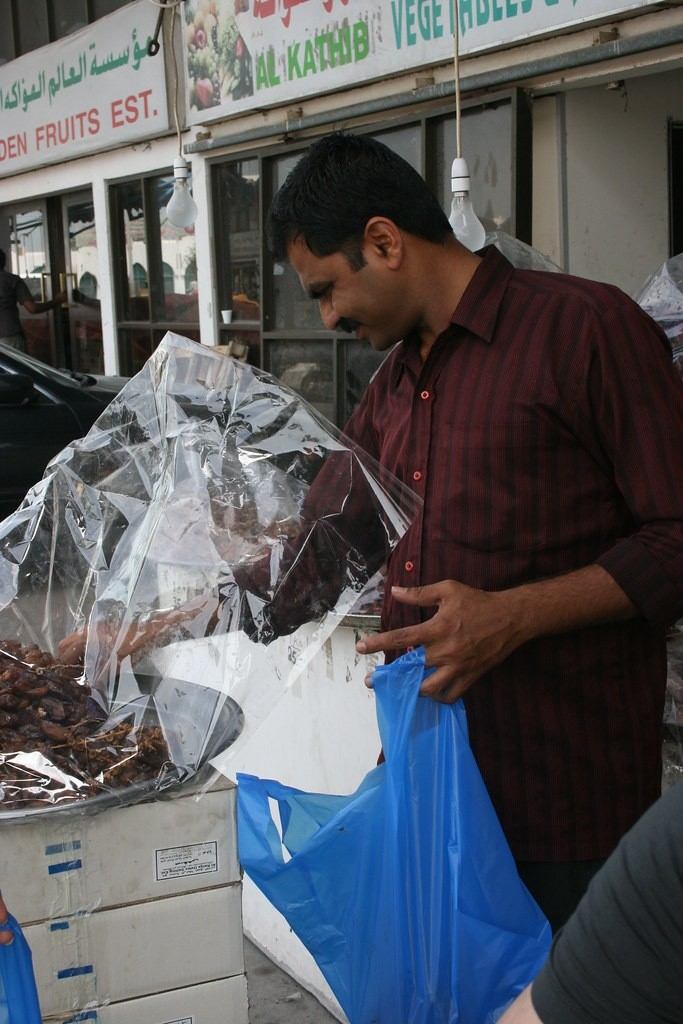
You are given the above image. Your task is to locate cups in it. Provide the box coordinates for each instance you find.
[221,310,232,324]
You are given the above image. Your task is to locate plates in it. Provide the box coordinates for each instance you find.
[1,671,242,825]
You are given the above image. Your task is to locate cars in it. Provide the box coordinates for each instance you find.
[0,341,131,520]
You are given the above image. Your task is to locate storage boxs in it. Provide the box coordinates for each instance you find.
[1,758,247,1024]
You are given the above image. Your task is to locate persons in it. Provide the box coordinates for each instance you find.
[57,128,681,1024]
[0,249,67,355]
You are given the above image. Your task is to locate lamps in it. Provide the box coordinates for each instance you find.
[447,1,488,252]
[163,0,199,228]
